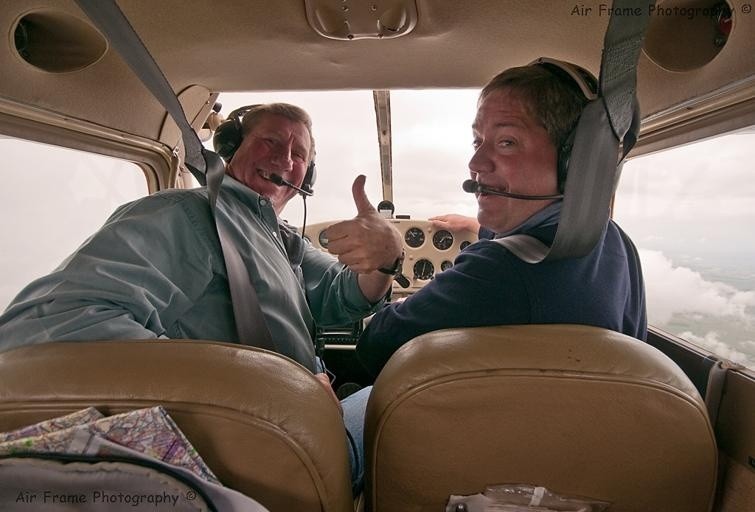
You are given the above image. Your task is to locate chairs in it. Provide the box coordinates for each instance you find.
[364,324,718,512]
[1,341,354,512]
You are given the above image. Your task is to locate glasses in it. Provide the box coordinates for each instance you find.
[526,57,600,102]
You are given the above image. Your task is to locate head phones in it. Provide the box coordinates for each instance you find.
[213,105,317,196]
[527,57,599,193]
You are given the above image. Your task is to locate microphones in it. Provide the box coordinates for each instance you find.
[269,174,314,195]
[462,180,564,200]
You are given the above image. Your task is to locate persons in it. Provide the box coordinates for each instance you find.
[1,101,405,503]
[354,59,648,373]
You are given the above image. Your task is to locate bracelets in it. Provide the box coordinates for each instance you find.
[378,248,406,278]
